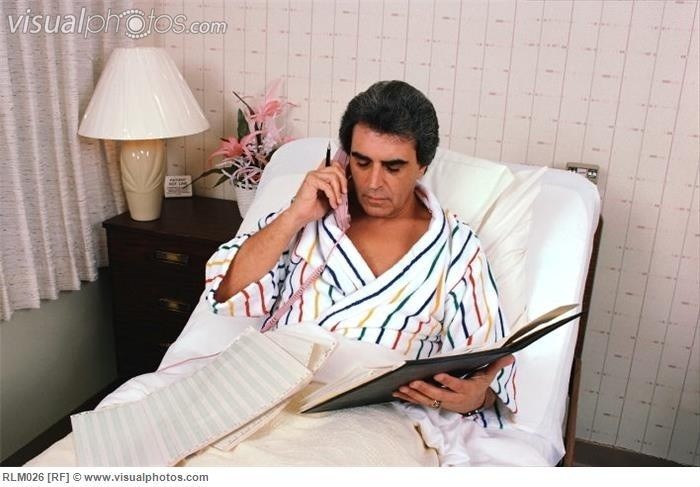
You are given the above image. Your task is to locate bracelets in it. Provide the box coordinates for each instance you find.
[462,396,486,416]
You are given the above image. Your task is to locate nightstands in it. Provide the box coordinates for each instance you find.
[102,196,243,379]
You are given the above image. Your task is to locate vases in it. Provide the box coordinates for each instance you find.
[232,184,254,219]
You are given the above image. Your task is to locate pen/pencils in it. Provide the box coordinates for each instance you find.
[326,139,331,168]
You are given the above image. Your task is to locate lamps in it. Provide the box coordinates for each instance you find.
[76,44,211,223]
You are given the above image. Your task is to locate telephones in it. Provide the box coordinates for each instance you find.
[331,147,351,231]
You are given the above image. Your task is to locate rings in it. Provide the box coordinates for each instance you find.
[432,399,442,409]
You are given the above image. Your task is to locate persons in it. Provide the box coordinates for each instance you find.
[201,81,516,433]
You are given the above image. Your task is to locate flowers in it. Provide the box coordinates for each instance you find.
[181,76,300,187]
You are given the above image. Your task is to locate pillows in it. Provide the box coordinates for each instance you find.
[417,146,512,237]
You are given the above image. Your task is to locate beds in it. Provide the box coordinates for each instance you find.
[20,138,604,467]
